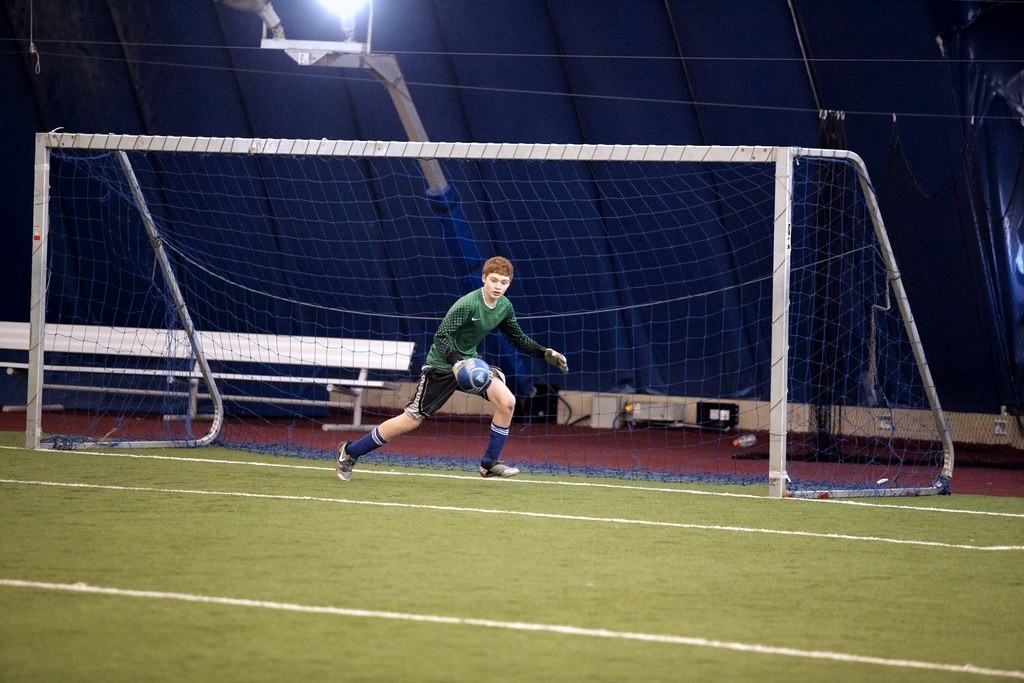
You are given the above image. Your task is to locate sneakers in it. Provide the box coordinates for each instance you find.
[338,441,355,482]
[481,458,520,478]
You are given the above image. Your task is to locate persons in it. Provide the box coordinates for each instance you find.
[336,256,569,481]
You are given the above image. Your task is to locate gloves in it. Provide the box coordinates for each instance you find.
[544,348,570,375]
[452,360,466,379]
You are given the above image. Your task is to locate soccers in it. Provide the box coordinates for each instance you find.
[455,357,494,394]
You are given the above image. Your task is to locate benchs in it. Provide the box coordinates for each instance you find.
[0,321,416,430]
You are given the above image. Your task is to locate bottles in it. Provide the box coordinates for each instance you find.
[733,434,757,448]
